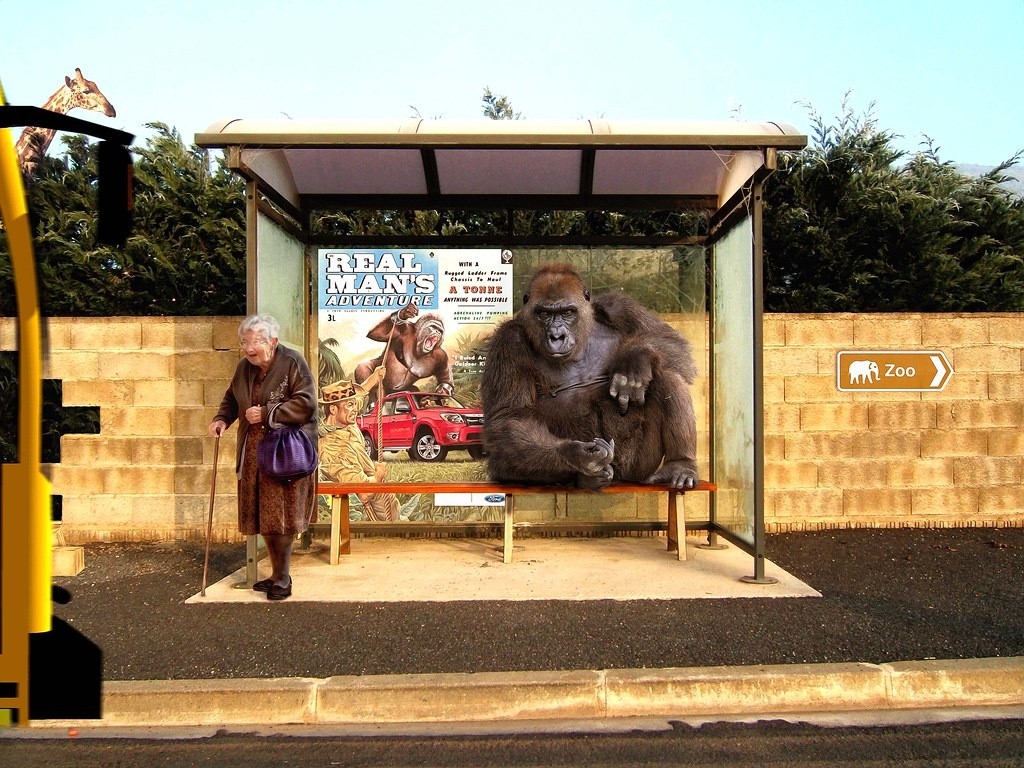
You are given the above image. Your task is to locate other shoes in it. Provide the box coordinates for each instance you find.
[253,579,272,591]
[267,575,291,600]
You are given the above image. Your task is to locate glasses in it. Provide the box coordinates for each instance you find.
[239,336,273,349]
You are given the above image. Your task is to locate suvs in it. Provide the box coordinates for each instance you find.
[355,391,484,462]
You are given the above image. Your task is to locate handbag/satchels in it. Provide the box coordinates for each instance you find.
[256,404,317,477]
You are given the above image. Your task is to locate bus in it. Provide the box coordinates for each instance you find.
[0,81,135,728]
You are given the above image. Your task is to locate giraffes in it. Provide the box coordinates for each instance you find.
[13,66,117,174]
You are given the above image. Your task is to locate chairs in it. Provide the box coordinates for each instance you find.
[397,403,409,414]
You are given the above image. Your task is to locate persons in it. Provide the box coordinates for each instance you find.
[209,314,319,601]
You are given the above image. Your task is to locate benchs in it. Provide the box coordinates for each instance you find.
[318,479,718,563]
